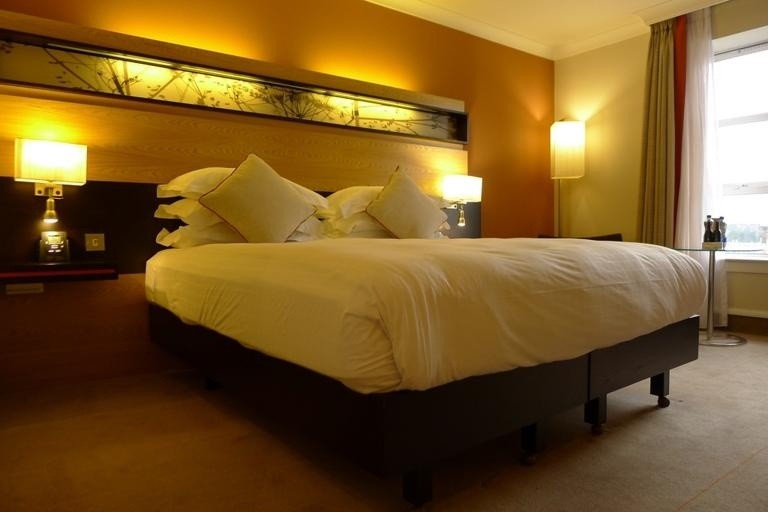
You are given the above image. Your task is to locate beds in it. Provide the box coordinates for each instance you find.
[145,232,711,506]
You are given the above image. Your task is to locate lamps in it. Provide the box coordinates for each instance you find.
[441,173,483,227]
[546,116,588,236]
[11,135,90,225]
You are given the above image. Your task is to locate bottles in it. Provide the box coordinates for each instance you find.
[703,215,727,243]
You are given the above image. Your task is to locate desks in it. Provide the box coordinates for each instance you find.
[671,244,765,349]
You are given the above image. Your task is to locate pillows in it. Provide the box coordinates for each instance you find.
[155,153,451,252]
